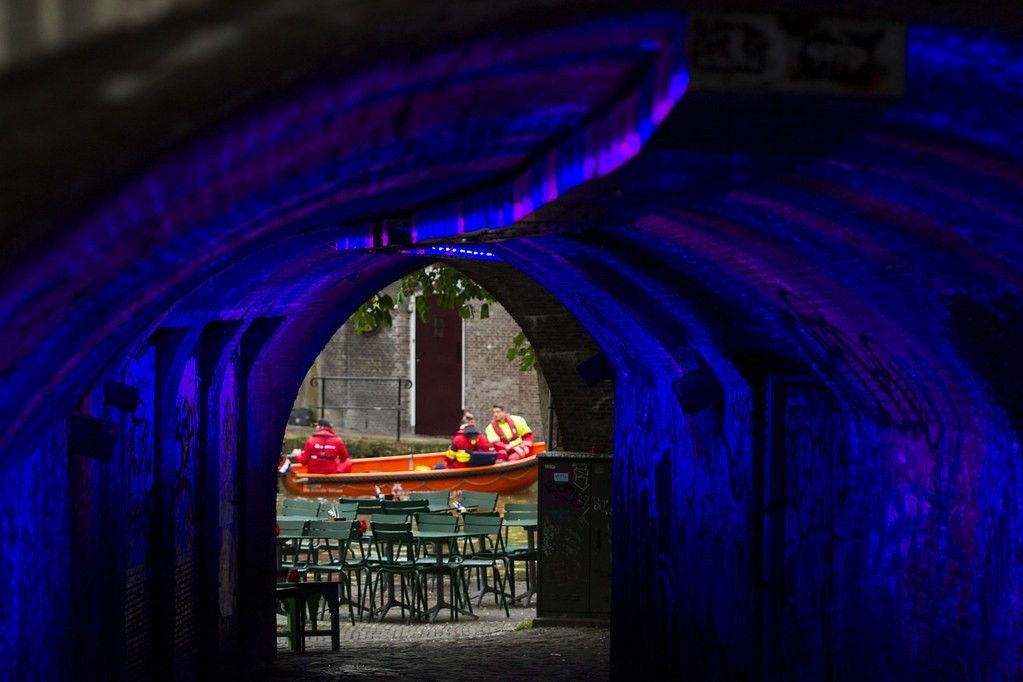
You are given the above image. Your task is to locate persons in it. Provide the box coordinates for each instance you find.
[444,411,490,469]
[293,419,352,475]
[483,402,533,464]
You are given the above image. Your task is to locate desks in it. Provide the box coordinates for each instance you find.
[317,526,488,625]
[453,518,540,608]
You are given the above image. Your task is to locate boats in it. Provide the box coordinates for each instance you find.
[279,442,546,499]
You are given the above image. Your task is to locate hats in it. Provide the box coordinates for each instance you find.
[312,420,329,426]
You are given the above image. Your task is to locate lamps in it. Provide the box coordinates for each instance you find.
[671,367,722,413]
[576,352,609,387]
[105,379,139,412]
[66,413,117,463]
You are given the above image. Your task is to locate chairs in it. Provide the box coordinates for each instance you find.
[273,491,540,656]
